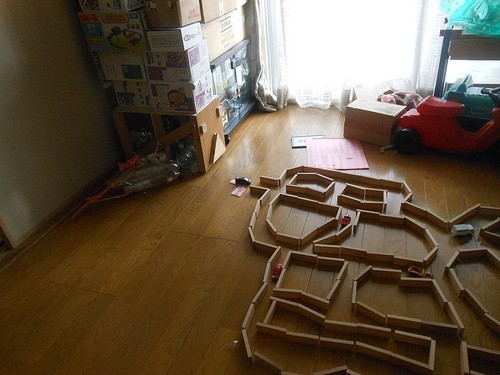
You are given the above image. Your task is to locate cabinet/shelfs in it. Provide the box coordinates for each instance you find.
[111,96,226,174]
[209,37,257,144]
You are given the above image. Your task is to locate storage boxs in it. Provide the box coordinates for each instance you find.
[344,98,407,147]
[69,0,261,114]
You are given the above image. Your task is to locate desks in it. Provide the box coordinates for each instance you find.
[433,17,500,98]
